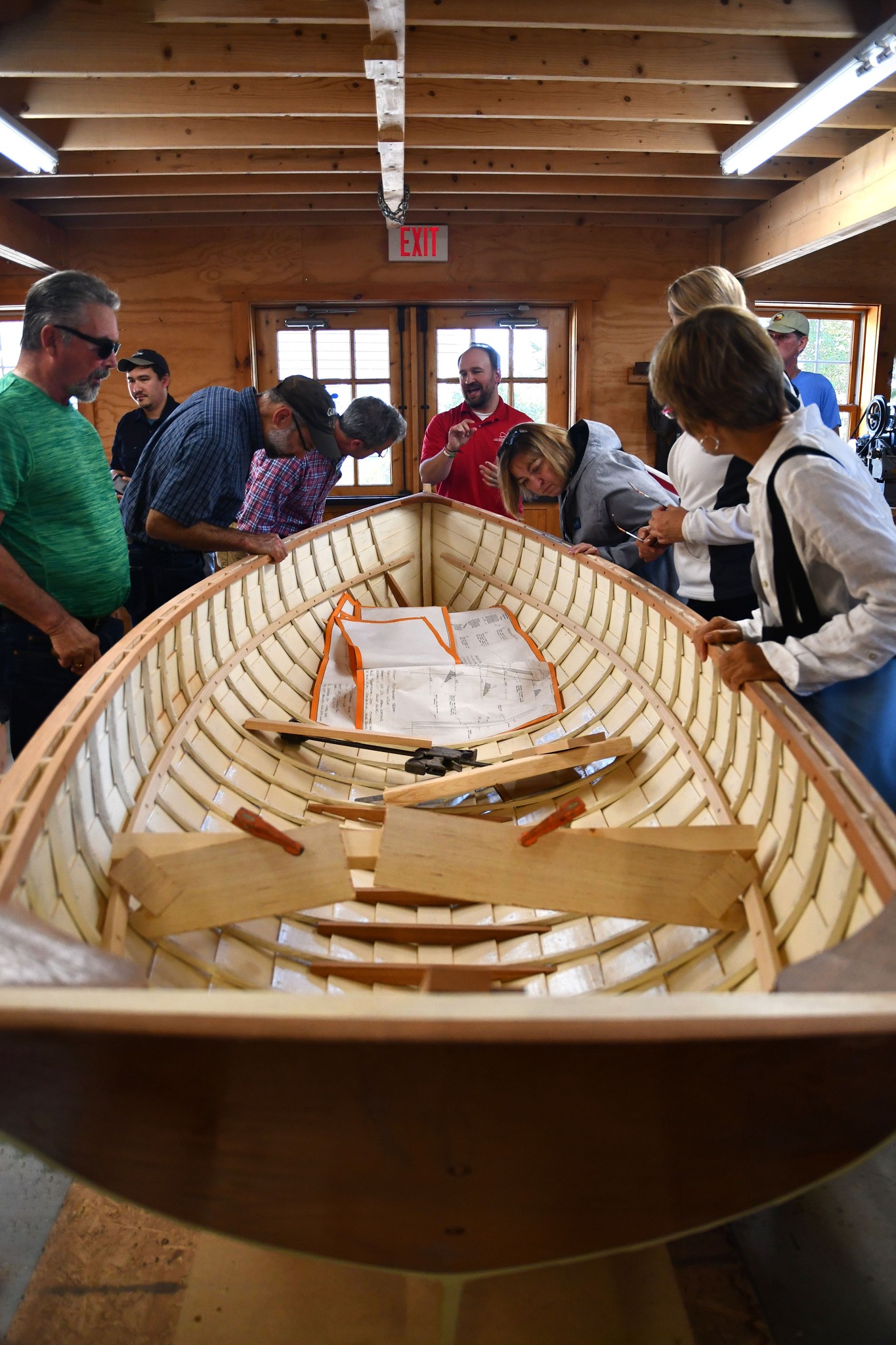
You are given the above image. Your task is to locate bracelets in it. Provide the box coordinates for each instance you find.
[443,451,457,459]
[444,443,460,453]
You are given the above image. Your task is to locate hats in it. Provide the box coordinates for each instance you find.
[118,349,169,376]
[276,375,342,459]
[767,310,809,336]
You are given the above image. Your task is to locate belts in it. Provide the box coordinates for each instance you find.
[77,616,110,631]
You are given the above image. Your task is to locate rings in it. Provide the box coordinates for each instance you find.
[73,664,82,668]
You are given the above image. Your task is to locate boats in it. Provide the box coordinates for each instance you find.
[0,478,895,1283]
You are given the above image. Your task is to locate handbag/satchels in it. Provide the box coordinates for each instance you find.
[760,444,896,816]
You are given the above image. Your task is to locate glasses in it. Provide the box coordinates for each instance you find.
[54,325,121,359]
[661,404,687,419]
[367,444,388,458]
[497,429,529,458]
[291,413,316,452]
[612,481,667,547]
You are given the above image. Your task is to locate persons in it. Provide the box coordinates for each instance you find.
[647,305,896,806]
[497,416,695,611]
[215,394,407,571]
[120,374,342,623]
[760,310,841,439]
[418,341,535,522]
[109,349,214,549]
[634,265,804,619]
[0,271,132,763]
